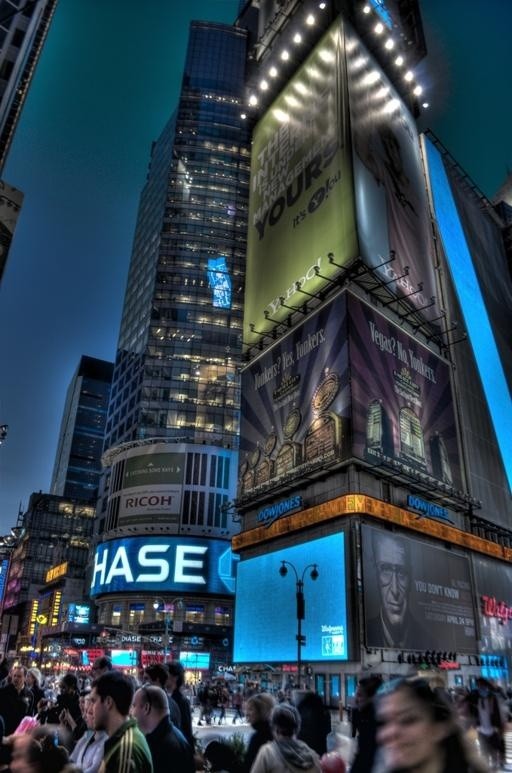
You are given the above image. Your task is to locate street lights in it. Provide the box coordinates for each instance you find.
[149,594,186,662]
[278,560,320,693]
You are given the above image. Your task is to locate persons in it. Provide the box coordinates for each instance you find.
[367,530,439,649]
